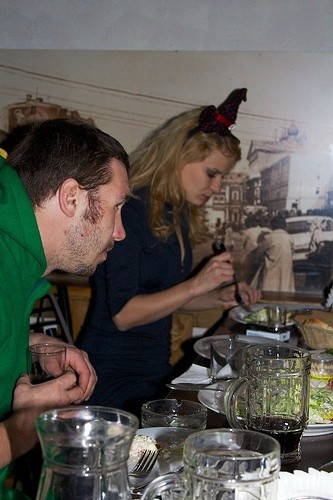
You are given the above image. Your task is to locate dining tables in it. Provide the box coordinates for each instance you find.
[143,298,333,478]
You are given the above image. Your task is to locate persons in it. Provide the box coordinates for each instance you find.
[223,220,236,251]
[249,217,296,292]
[308,218,328,254]
[0,116,127,500]
[70,87,261,425]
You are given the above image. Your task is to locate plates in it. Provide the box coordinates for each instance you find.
[194,334,287,362]
[127,426,199,489]
[197,381,333,436]
[229,303,324,328]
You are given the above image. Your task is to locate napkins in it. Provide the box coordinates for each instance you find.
[169,362,237,387]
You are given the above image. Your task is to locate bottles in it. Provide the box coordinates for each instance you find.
[34,405,138,500]
[310,354,333,380]
[139,399,207,435]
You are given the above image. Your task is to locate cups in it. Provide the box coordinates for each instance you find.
[26,343,67,384]
[141,427,281,500]
[267,305,285,334]
[225,344,313,462]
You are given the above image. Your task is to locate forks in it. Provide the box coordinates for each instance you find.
[128,447,160,480]
[219,242,252,312]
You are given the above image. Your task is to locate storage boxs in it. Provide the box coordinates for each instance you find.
[246,330,292,343]
